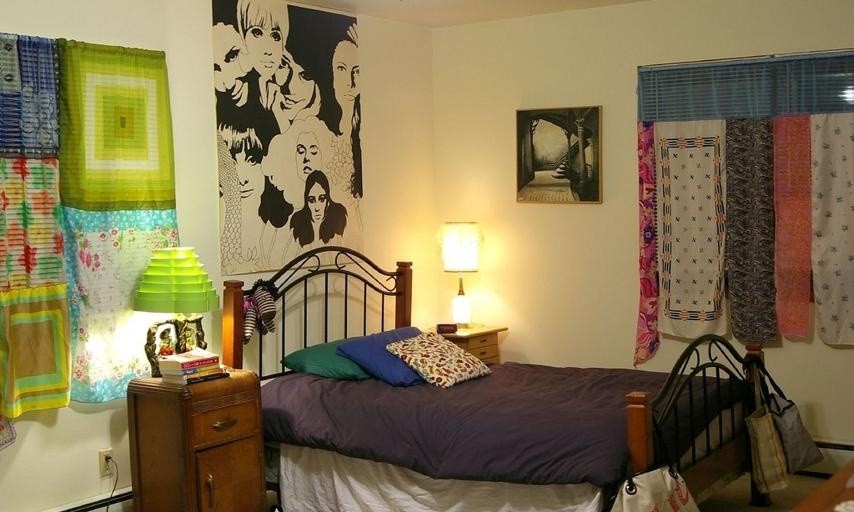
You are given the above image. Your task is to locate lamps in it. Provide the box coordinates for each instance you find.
[441,221,482,328]
[129,246,222,355]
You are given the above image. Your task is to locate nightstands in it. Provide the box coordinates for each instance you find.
[128,368,268,512]
[425,327,509,370]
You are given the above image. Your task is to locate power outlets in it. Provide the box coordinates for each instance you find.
[100,449,115,478]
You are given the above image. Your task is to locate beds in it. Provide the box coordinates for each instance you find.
[225,244,763,512]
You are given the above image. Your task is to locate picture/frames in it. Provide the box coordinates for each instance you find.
[515,104,605,206]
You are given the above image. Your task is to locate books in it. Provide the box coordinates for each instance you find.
[159,347,230,385]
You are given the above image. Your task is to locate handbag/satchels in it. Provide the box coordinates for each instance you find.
[743,393,824,494]
[609,466,700,512]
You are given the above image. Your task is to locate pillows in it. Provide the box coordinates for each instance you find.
[280,326,492,391]
